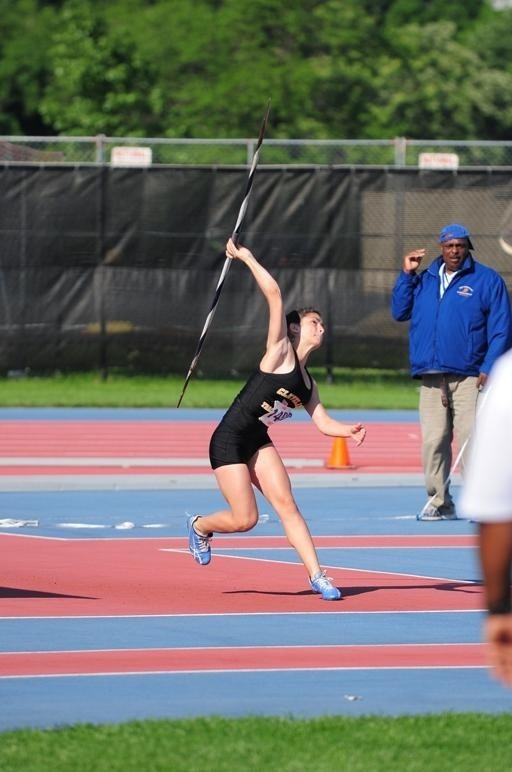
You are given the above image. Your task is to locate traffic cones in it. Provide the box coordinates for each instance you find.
[324,437,356,469]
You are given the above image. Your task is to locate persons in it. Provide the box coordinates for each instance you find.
[454,345,512,694]
[388,222,512,525]
[186,237,369,604]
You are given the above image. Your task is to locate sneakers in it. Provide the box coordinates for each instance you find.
[307,569,341,601]
[417,505,457,521]
[186,514,213,565]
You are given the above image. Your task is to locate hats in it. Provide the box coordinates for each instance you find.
[439,224,475,252]
